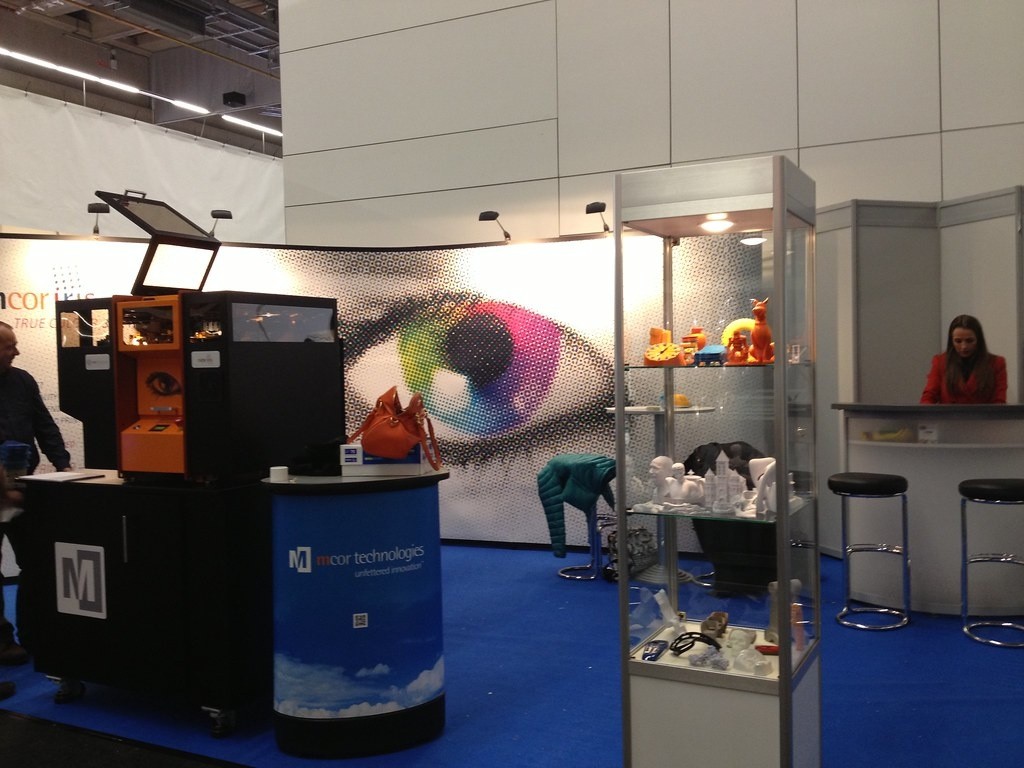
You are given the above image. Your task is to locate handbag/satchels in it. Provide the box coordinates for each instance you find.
[608,528,658,577]
[346,384,440,471]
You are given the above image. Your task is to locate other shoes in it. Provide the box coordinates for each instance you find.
[0,644,29,666]
[0,682,16,700]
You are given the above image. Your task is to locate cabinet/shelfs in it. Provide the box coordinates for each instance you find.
[610,153,826,768]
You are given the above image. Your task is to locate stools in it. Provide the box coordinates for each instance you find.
[828,472,912,630]
[957,478,1024,647]
[551,454,613,580]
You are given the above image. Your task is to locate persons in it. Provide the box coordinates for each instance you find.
[650,456,702,503]
[920,314,1008,404]
[0,321,74,665]
